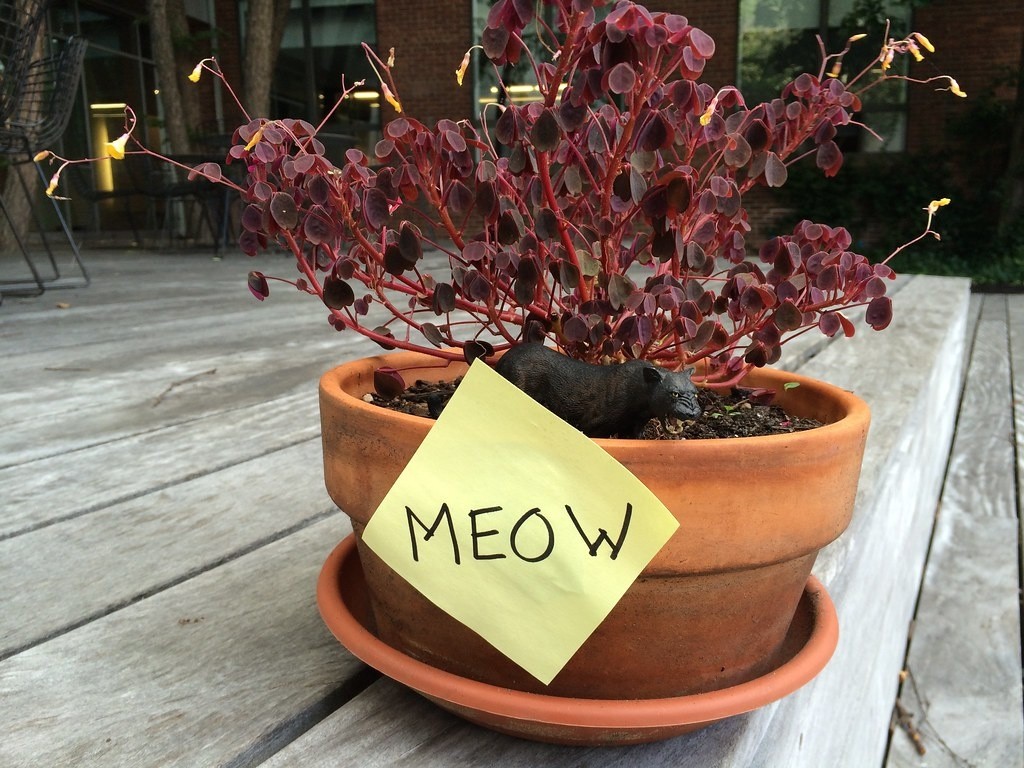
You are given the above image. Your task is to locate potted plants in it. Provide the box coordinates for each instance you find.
[209,16,944,745]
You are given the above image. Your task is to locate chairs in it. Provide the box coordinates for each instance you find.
[0,0,246,295]
